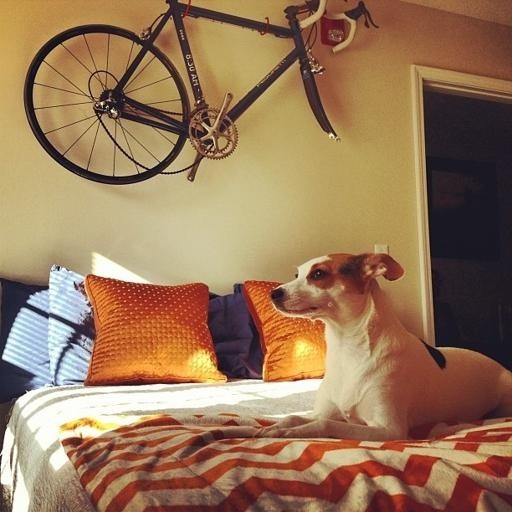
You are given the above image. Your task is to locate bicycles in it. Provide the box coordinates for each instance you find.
[24,1,379,186]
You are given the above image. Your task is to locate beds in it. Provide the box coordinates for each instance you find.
[1,264,511,512]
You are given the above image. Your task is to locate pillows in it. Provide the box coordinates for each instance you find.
[82,273,228,386]
[241,280,327,383]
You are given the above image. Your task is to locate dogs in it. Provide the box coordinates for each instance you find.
[248,249,512,441]
